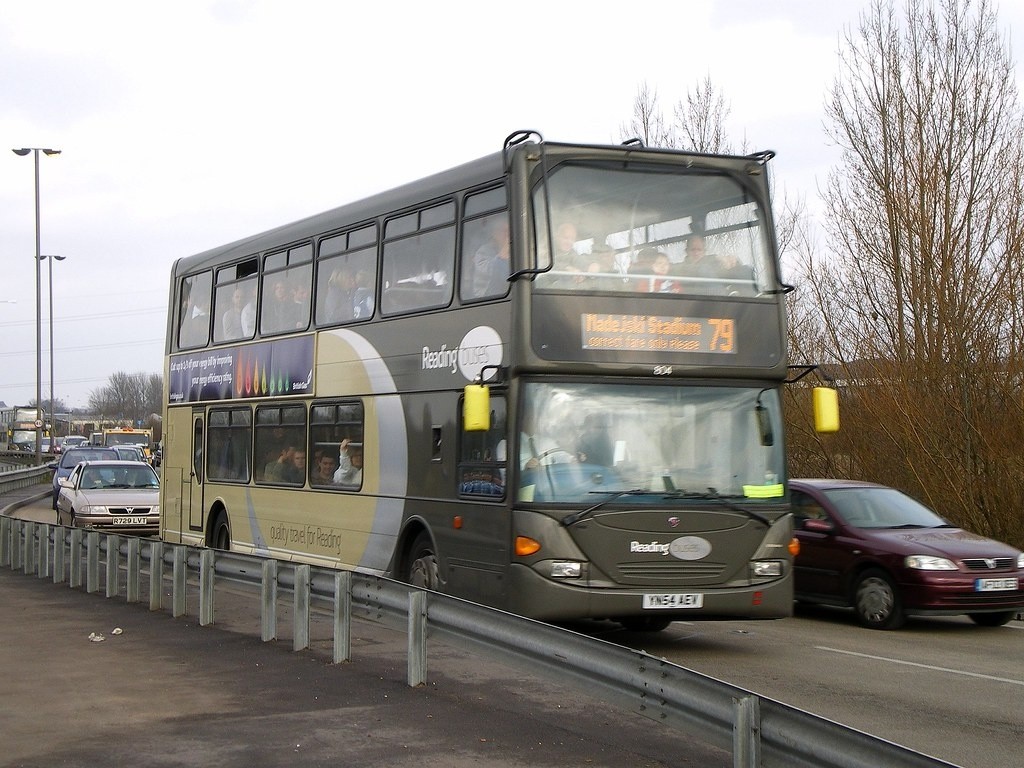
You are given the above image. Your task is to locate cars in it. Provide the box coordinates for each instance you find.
[57,459,162,534]
[46,446,130,510]
[786,479,1024,632]
[40,427,163,468]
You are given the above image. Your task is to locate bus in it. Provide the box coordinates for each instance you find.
[2,405,51,455]
[166,128,844,659]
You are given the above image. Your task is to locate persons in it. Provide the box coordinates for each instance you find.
[197,406,361,498]
[794,496,824,531]
[497,405,612,487]
[459,473,508,496]
[189,214,743,344]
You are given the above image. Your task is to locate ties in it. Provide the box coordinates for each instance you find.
[529,438,540,465]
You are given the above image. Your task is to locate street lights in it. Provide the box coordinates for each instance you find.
[33,253,67,464]
[11,147,62,464]
